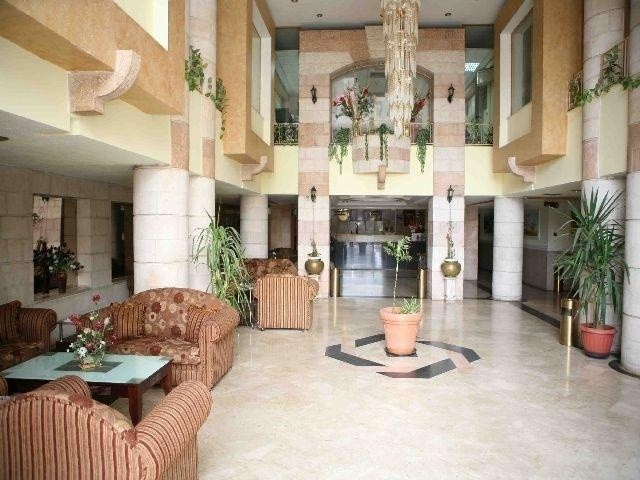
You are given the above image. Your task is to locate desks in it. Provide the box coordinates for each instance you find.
[3,352,174,426]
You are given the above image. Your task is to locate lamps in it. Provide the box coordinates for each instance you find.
[309,84,317,104]
[378,0,420,142]
[446,184,455,204]
[310,186,317,201]
[446,83,455,103]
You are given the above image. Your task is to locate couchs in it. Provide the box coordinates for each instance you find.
[0,374,213,480]
[1,300,57,370]
[75,288,240,389]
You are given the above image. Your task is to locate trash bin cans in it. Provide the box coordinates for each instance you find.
[330,267,340,297]
[553,266,564,293]
[417,268,427,299]
[560,298,580,347]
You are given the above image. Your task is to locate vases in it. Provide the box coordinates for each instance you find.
[73,350,105,369]
[48,270,68,294]
[350,114,362,137]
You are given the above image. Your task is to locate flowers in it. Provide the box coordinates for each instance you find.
[42,241,84,274]
[66,293,117,369]
[333,86,376,117]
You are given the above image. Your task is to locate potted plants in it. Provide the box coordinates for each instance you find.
[379,236,425,356]
[547,186,631,359]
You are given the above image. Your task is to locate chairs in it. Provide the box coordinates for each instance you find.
[244,257,319,331]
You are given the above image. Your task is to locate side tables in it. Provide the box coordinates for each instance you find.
[57,314,81,351]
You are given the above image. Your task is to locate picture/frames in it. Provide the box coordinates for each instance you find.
[524,206,543,241]
